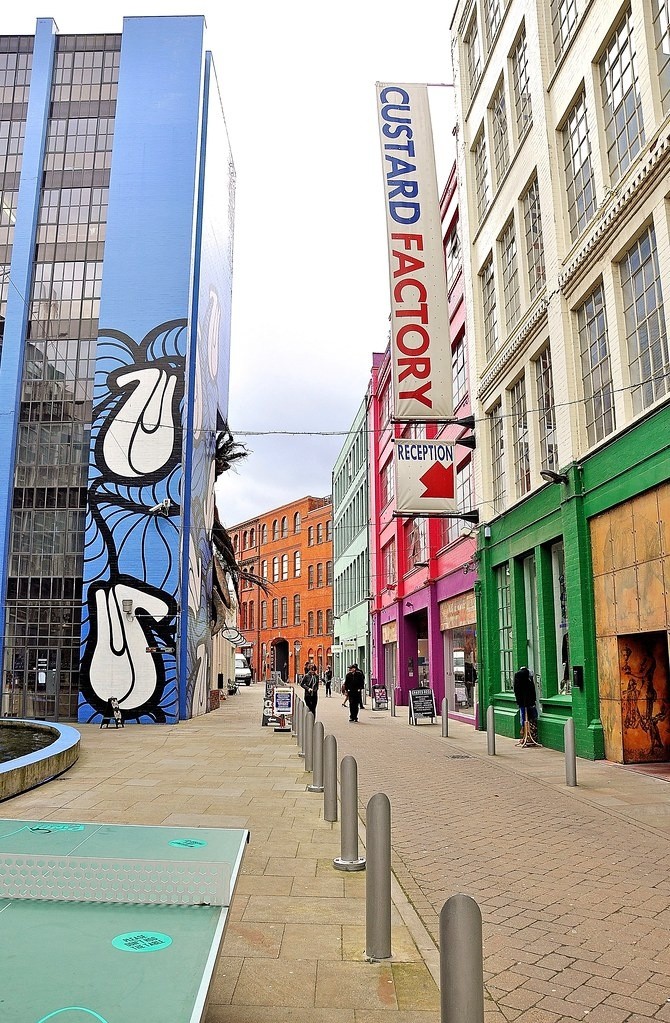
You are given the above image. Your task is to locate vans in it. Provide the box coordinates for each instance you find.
[234,653,252,686]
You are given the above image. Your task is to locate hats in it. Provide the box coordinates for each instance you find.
[348,664,357,669]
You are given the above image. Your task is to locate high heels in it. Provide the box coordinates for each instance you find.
[342,704,347,707]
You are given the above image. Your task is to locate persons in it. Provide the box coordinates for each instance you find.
[514,667,537,735]
[300,664,319,721]
[342,664,365,709]
[345,665,363,722]
[325,665,332,697]
[304,662,309,676]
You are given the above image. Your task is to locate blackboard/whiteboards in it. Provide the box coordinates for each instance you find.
[409,687,435,718]
[373,685,388,703]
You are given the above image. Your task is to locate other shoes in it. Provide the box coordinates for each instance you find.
[355,720,358,722]
[349,719,354,721]
[360,706,365,709]
